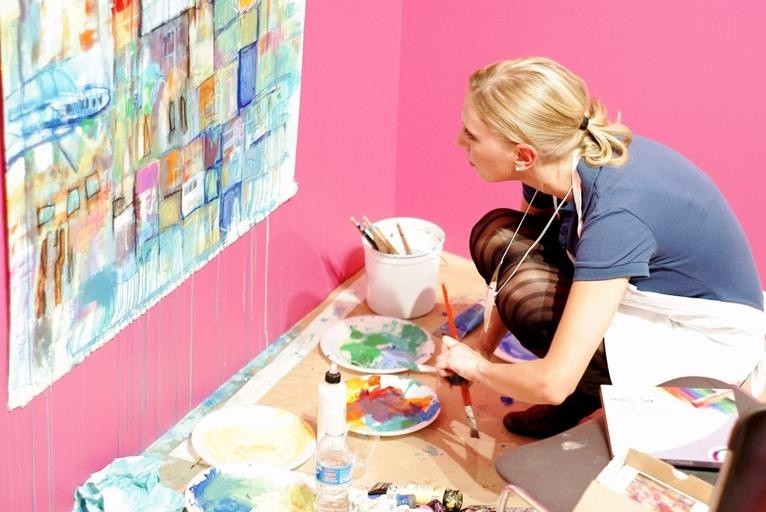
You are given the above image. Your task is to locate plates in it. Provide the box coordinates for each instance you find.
[184,463,312,511]
[318,314,436,376]
[342,371,444,437]
[488,329,542,365]
[189,402,314,478]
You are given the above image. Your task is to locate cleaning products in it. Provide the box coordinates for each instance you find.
[313,353,348,448]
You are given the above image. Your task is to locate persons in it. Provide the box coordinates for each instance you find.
[434,57,763,440]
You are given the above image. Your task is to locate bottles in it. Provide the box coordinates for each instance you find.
[318,359,350,444]
[312,420,357,512]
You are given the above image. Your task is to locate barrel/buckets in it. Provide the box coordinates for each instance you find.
[362,216,446,321]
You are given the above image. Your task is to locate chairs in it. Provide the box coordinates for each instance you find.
[494,376,766,512]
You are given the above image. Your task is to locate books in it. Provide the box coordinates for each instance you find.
[596,381,740,467]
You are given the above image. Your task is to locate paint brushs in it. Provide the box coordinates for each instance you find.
[442,282,480,440]
[350,215,413,255]
